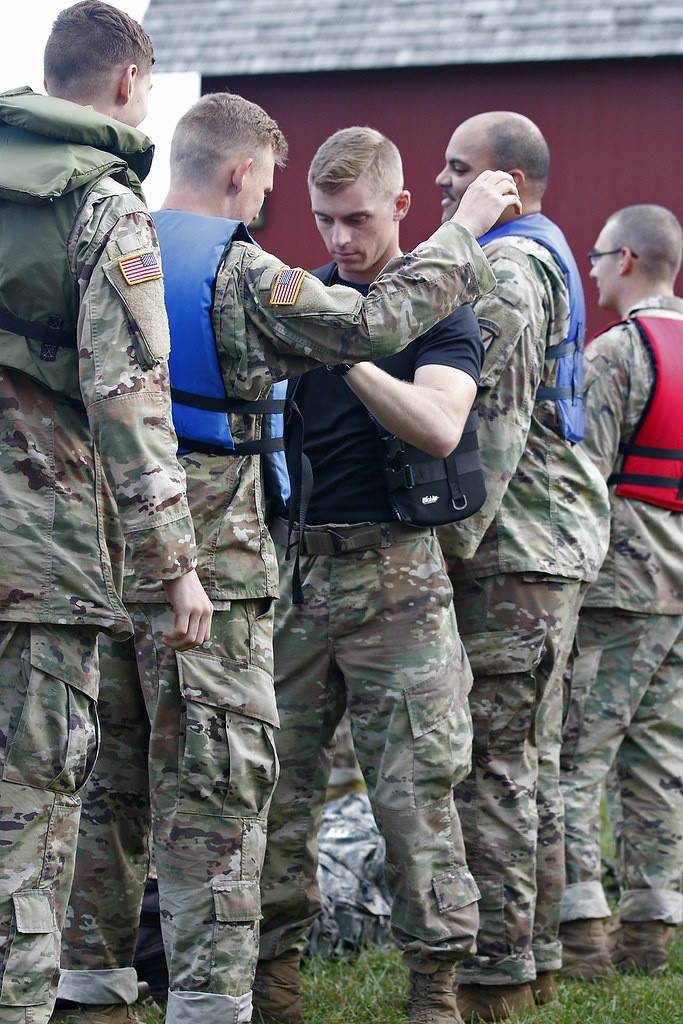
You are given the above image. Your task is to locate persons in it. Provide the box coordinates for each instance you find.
[0,0,683,1024]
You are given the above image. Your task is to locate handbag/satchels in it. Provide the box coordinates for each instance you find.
[381,409,487,529]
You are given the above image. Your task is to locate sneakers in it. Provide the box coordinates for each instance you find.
[457,971,559,1024]
[602,921,672,978]
[49,997,142,1024]
[404,960,464,1024]
[250,949,304,1024]
[554,918,617,981]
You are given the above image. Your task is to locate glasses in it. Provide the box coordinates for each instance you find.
[588,248,638,267]
